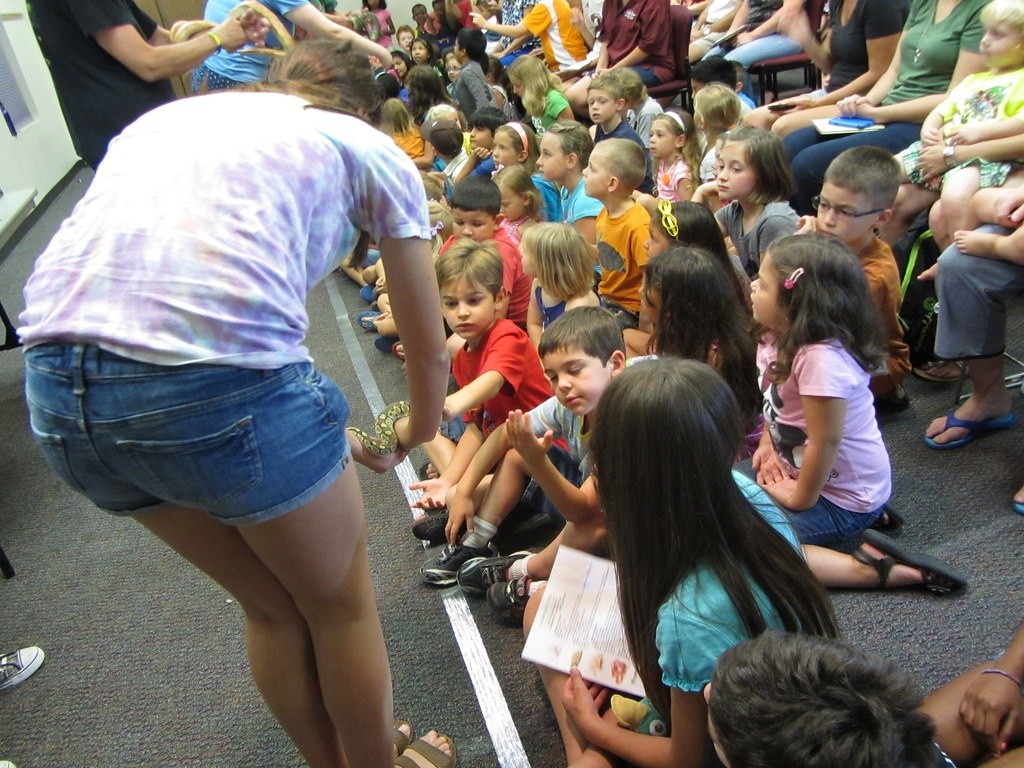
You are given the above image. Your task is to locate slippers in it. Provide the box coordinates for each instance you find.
[923,406,1018,450]
[912,359,972,383]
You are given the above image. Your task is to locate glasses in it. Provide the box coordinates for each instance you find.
[810,195,886,221]
[445,66,462,72]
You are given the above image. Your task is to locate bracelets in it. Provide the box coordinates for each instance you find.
[702,28,711,36]
[208,31,222,55]
[942,146,956,168]
[982,670,1021,686]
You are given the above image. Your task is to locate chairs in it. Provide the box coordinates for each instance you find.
[746,0,826,104]
[646,4,695,118]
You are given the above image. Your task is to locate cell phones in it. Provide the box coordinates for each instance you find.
[828,116,876,129]
[767,103,796,111]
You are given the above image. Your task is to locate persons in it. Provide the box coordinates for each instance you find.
[191,0,392,95]
[334,0,1024,768]
[16,37,456,768]
[26,0,271,174]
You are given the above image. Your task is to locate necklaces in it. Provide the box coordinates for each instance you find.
[913,13,933,63]
[507,215,530,237]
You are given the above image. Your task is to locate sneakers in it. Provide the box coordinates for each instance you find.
[0,646,45,692]
[456,549,533,596]
[419,534,500,586]
[485,573,540,624]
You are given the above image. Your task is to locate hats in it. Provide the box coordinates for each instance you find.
[420,118,464,155]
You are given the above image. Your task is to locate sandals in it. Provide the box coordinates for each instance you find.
[357,310,382,333]
[411,510,468,542]
[393,719,416,756]
[395,732,457,768]
[418,461,440,481]
[852,528,968,596]
[871,504,906,532]
[373,335,401,354]
[391,341,406,362]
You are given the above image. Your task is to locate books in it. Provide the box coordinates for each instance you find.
[811,118,885,135]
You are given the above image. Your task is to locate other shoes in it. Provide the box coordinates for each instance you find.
[359,284,378,304]
